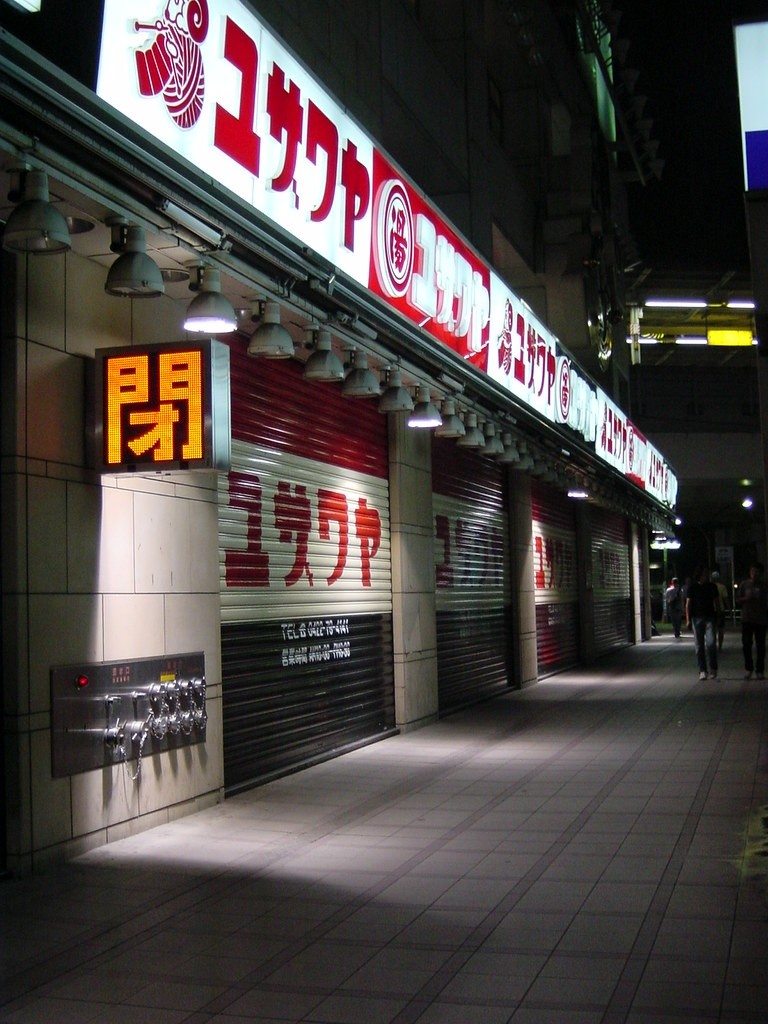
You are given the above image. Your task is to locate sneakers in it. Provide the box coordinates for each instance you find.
[709,667,719,679]
[699,672,708,680]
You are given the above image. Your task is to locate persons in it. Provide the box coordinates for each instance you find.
[665,569,733,680]
[735,561,768,680]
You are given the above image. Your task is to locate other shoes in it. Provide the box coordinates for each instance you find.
[744,670,765,680]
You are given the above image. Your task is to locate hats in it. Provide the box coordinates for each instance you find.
[712,571,719,578]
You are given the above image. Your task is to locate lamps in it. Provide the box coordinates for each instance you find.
[0,165,73,257]
[183,265,238,334]
[302,326,344,382]
[497,433,520,464]
[247,298,294,360]
[105,218,164,299]
[476,422,505,454]
[375,365,414,413]
[512,441,534,471]
[407,381,442,427]
[530,446,681,549]
[457,412,485,449]
[432,397,465,438]
[340,345,382,397]
